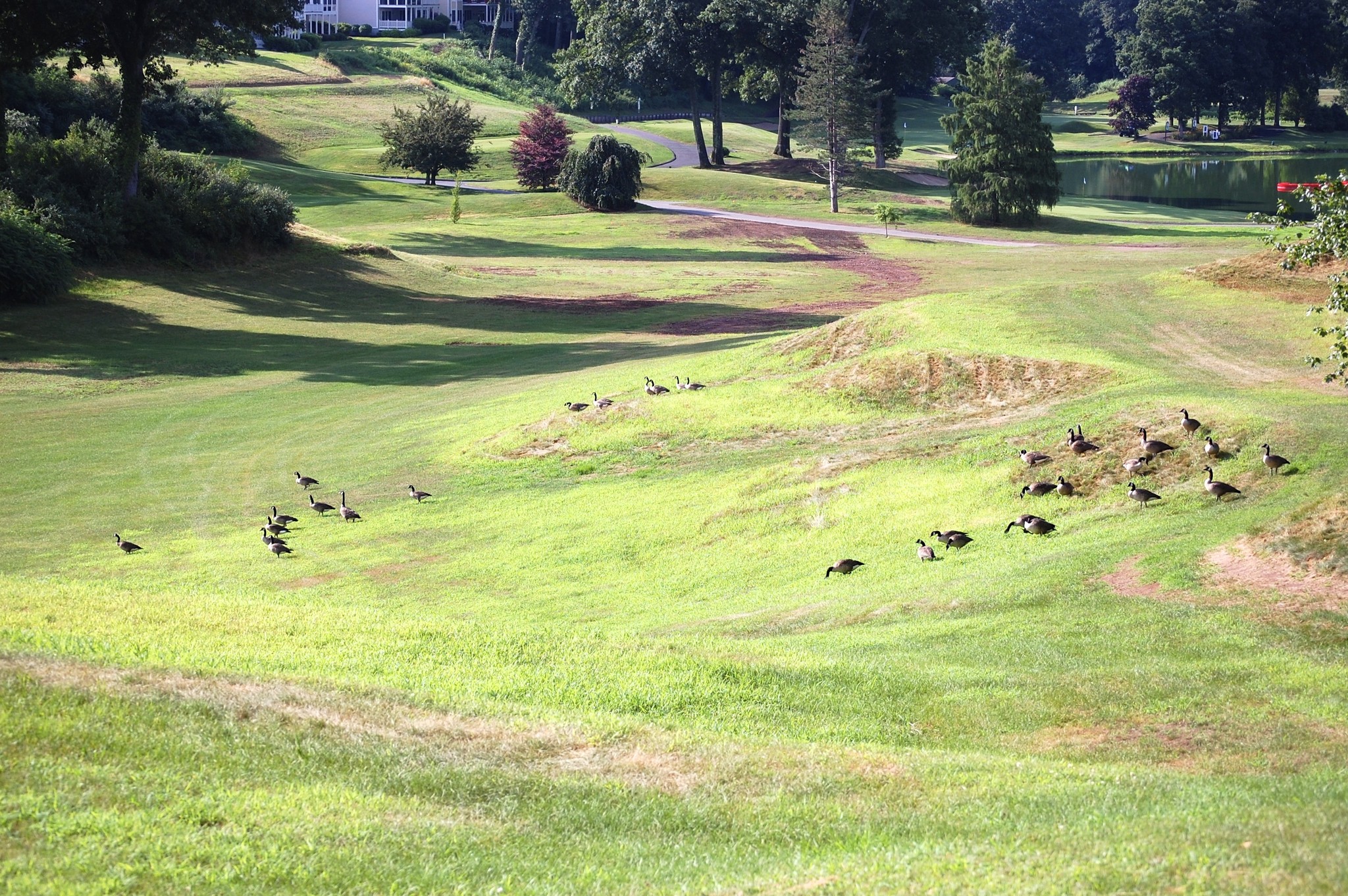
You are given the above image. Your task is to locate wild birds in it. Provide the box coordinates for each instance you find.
[113,534,143,554]
[1056,476,1075,498]
[260,506,299,558]
[1179,408,1201,439]
[1067,428,1099,457]
[408,485,432,504]
[1137,427,1174,458]
[293,471,361,523]
[1203,467,1241,502]
[564,392,614,412]
[1020,482,1057,499]
[1022,517,1057,537]
[1018,449,1051,468]
[1261,444,1290,476]
[946,534,975,551]
[643,376,707,396]
[1004,515,1045,534]
[1204,437,1219,459]
[1142,451,1153,466]
[929,530,968,544]
[915,539,935,562]
[1123,456,1148,477]
[824,559,865,579]
[1127,482,1162,510]
[1067,424,1084,446]
